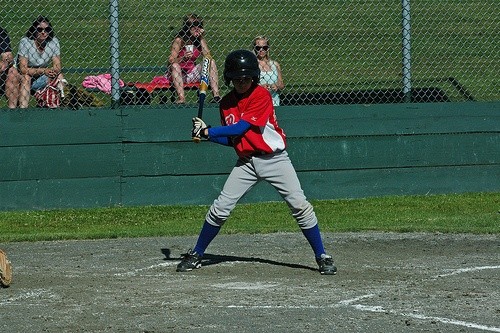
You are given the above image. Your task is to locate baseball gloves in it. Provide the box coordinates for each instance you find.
[0,249,13,287]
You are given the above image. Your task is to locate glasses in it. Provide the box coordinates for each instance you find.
[255,46,268,51]
[37,27,51,32]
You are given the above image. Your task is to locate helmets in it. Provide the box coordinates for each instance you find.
[223,50,260,87]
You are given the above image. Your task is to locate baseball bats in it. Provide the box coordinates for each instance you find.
[190,53,212,143]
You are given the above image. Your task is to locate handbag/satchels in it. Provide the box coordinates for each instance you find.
[34,74,60,108]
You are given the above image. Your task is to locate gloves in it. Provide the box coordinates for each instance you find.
[192,117,211,141]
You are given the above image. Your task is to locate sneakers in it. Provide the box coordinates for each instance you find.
[317,254,337,275]
[177,248,202,272]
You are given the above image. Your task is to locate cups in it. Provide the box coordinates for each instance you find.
[185,45,195,53]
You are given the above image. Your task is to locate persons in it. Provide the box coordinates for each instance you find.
[251,36,284,106]
[169,13,222,105]
[176,50,339,275]
[17,16,65,109]
[0,26,18,108]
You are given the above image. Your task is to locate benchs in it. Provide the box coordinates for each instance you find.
[62,67,212,91]
